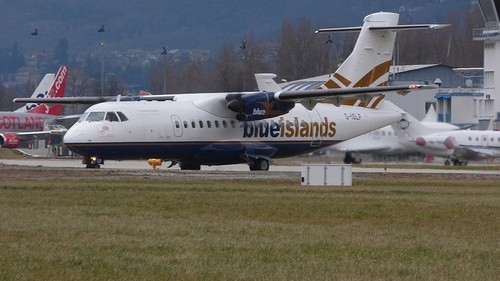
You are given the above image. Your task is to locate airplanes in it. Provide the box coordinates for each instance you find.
[331,105,461,165]
[14,10,452,172]
[0,65,88,149]
[379,101,500,166]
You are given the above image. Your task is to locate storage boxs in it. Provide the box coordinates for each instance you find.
[299,164,354,187]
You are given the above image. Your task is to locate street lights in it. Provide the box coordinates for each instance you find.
[98,23,106,96]
[29,28,40,90]
[239,40,248,92]
[161,46,167,94]
[325,33,334,79]
